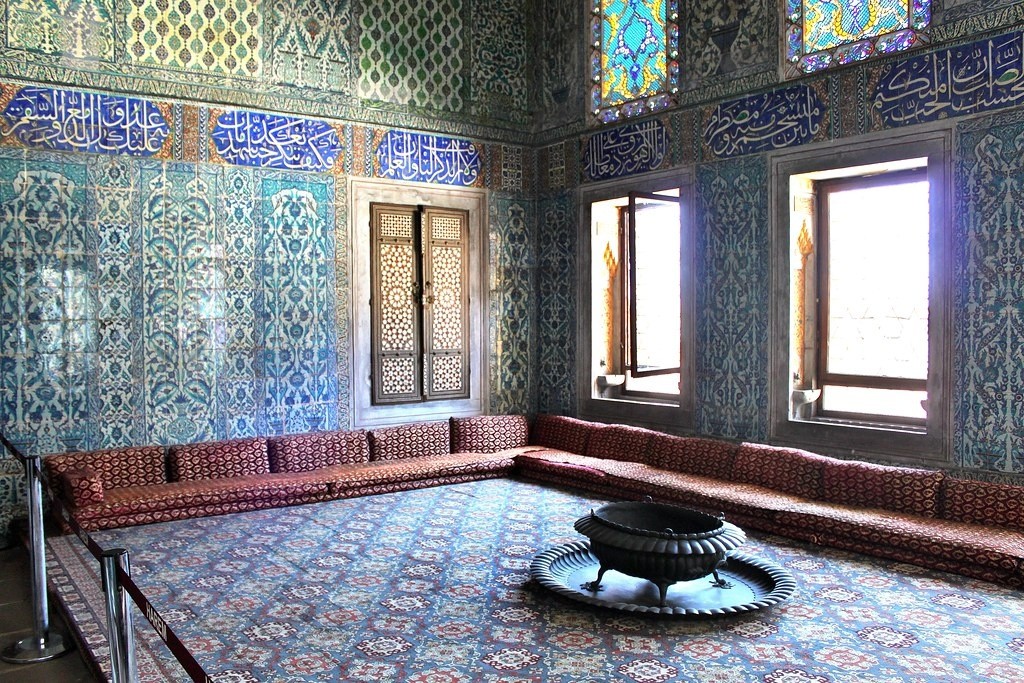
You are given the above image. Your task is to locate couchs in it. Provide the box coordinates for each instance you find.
[37,408,1024,585]
